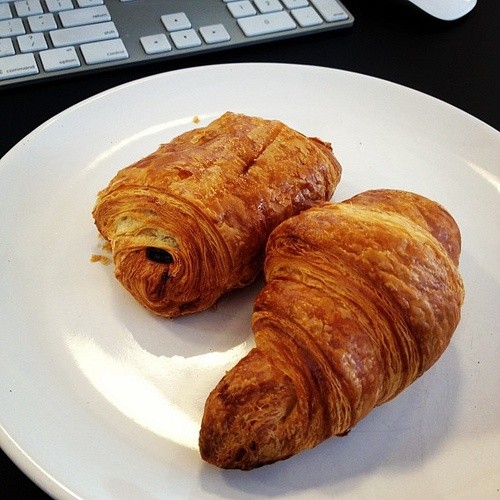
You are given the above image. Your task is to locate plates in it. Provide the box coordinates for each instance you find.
[0,61,500,500]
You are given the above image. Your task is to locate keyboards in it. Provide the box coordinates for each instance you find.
[0,0,355,92]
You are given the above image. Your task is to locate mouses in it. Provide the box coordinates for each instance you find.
[409,0,478,22]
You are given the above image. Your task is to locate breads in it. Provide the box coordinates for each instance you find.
[90,110,343,318]
[197,187,468,474]
